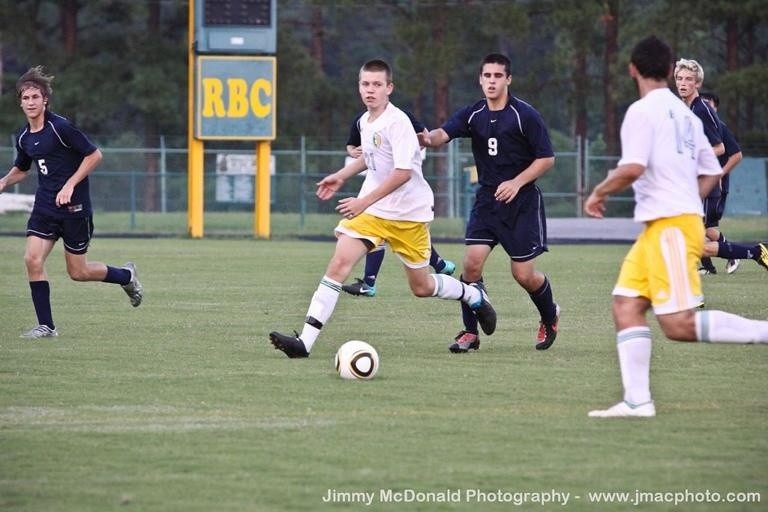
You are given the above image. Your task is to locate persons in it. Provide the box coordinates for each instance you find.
[696,93,741,275]
[416,52,561,354]
[342,143,455,297]
[0,62,144,337]
[675,54,768,308]
[269,59,497,358]
[582,33,768,418]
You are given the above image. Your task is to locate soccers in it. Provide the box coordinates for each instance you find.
[335,340,379,379]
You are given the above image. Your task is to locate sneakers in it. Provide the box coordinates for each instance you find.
[121,262,143,307]
[468,281,496,335]
[342,280,376,296]
[698,267,716,275]
[20,324,58,339]
[726,259,741,273]
[756,241,768,270]
[437,260,455,275]
[536,303,560,349]
[269,332,309,358]
[449,331,479,353]
[589,400,656,416]
